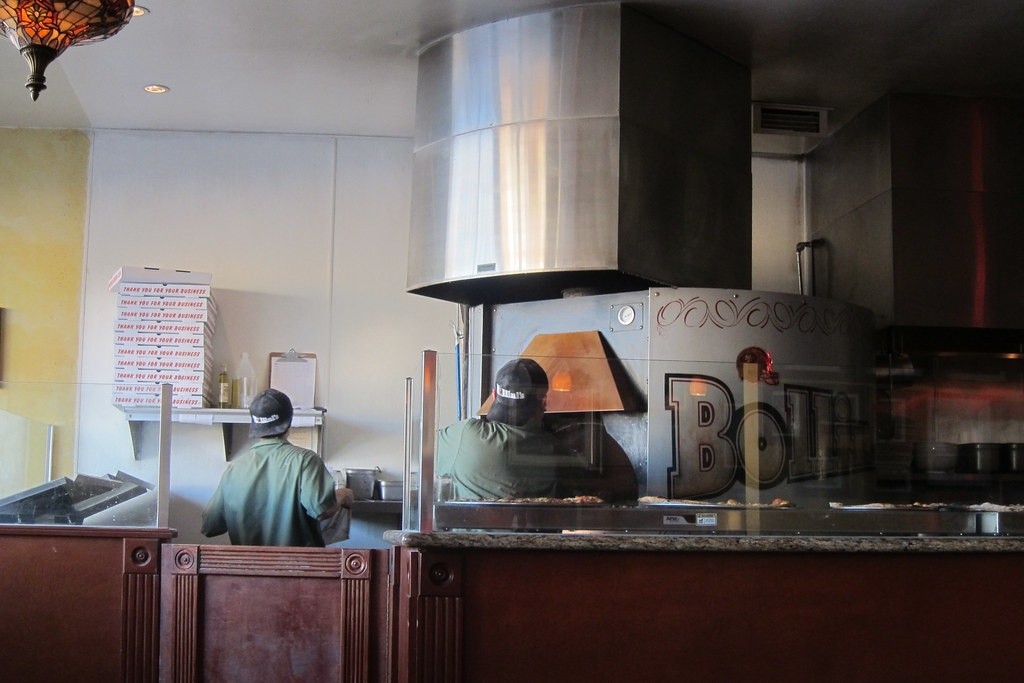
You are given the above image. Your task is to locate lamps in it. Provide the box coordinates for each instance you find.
[0,0,135,101]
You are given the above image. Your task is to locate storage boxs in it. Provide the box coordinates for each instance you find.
[106,265,217,409]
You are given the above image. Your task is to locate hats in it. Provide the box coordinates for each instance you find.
[486,358,548,426]
[249,389,292,437]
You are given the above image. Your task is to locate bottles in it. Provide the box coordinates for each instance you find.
[234,352,256,408]
[216,363,231,408]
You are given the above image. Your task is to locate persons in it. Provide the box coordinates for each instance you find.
[434,358,589,532]
[199,389,354,548]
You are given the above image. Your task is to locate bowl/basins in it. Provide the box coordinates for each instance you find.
[874,415,1024,473]
